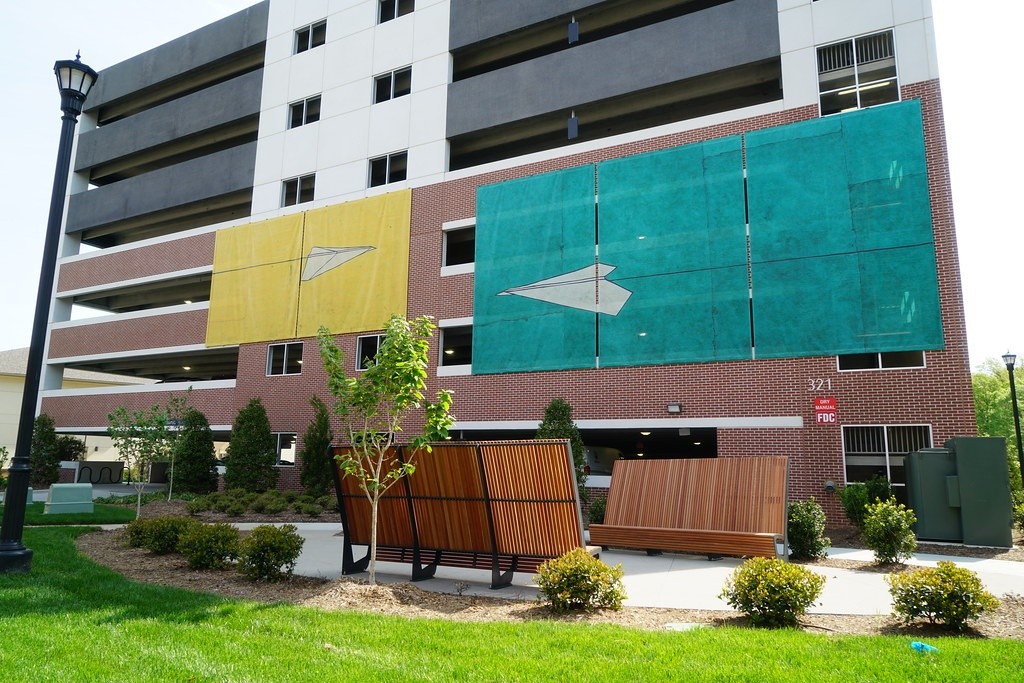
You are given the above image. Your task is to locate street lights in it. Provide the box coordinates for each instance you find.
[1001,349,1024,487]
[0,50,99,573]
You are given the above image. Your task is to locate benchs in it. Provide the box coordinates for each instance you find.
[328,438,602,590]
[588,456,789,564]
